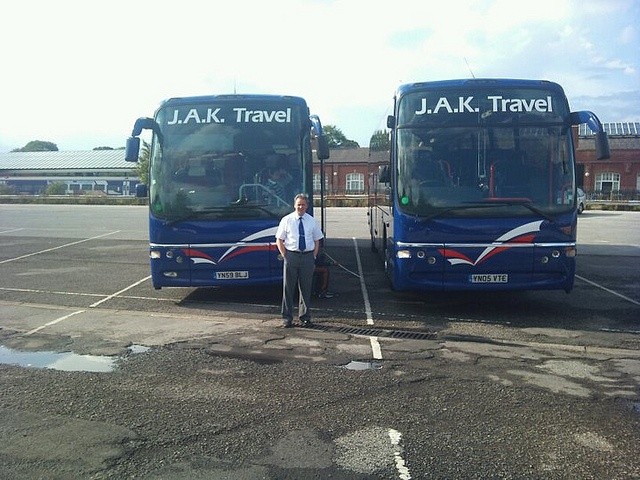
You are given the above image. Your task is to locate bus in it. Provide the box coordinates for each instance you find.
[368,78,609,291]
[124,94,329,289]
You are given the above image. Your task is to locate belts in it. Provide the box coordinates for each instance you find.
[290,249,315,254]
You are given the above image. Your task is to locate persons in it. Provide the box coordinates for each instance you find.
[274,193,325,328]
[263,167,286,207]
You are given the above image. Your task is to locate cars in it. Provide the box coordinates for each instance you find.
[577,188,586,214]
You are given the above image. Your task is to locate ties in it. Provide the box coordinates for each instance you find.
[299,217,306,252]
